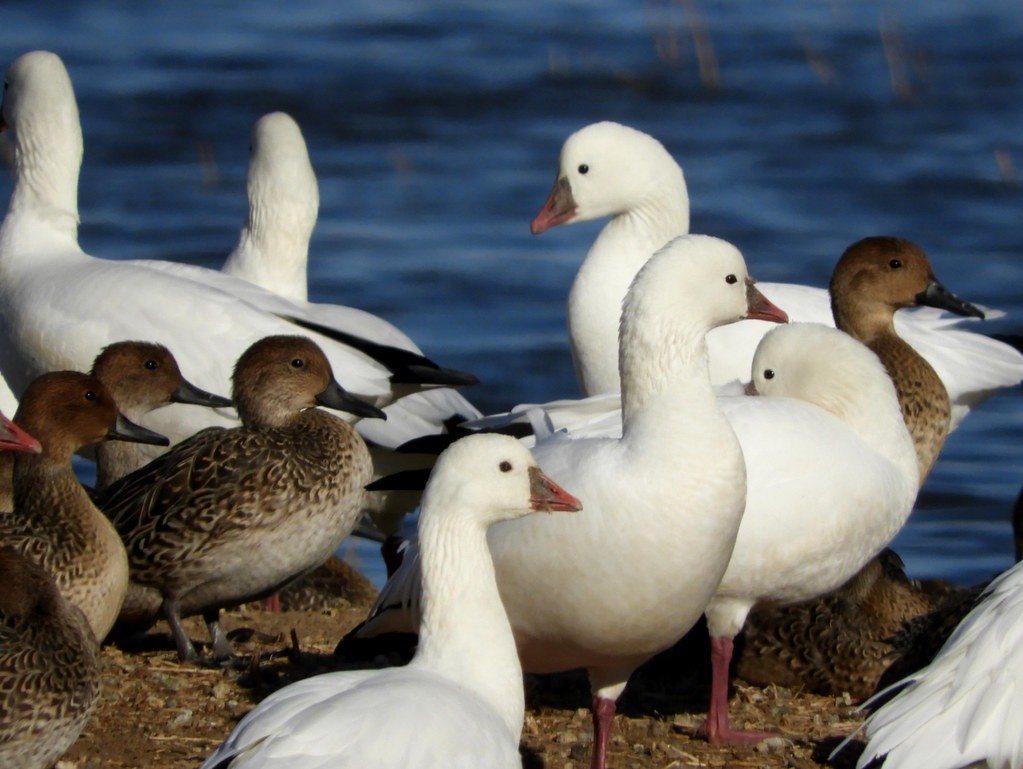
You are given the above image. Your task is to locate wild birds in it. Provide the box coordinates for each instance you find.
[5,370,170,645]
[222,111,541,580]
[362,322,919,750]
[69,341,383,614]
[2,49,488,461]
[532,122,1023,432]
[332,236,787,762]
[0,411,105,769]
[197,430,582,769]
[823,486,1022,769]
[90,337,388,665]
[745,236,987,482]
